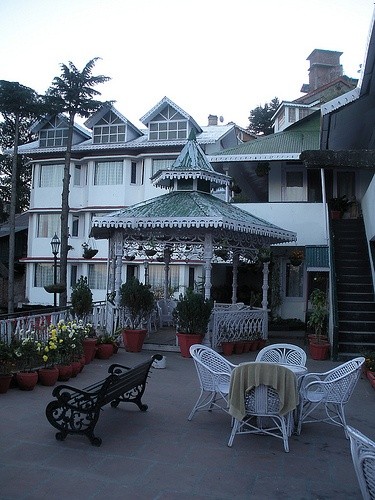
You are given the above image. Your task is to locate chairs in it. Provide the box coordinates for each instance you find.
[255,343,307,421]
[344,424,375,500]
[187,343,238,427]
[299,356,366,438]
[227,361,298,454]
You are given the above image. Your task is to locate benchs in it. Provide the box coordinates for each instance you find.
[45,352,164,447]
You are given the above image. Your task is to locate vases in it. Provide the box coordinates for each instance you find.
[221,339,266,355]
[0,355,86,392]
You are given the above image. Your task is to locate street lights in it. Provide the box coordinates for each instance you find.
[50,231,61,307]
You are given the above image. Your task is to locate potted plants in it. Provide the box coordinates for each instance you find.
[331,198,346,219]
[308,289,329,361]
[71,275,155,364]
[290,252,304,267]
[362,350,375,387]
[258,244,271,263]
[81,242,156,260]
[176,290,211,358]
[215,240,230,257]
[255,162,270,176]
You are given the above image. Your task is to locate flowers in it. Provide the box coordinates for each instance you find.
[0,318,95,376]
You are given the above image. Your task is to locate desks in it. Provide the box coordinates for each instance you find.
[256,362,307,430]
